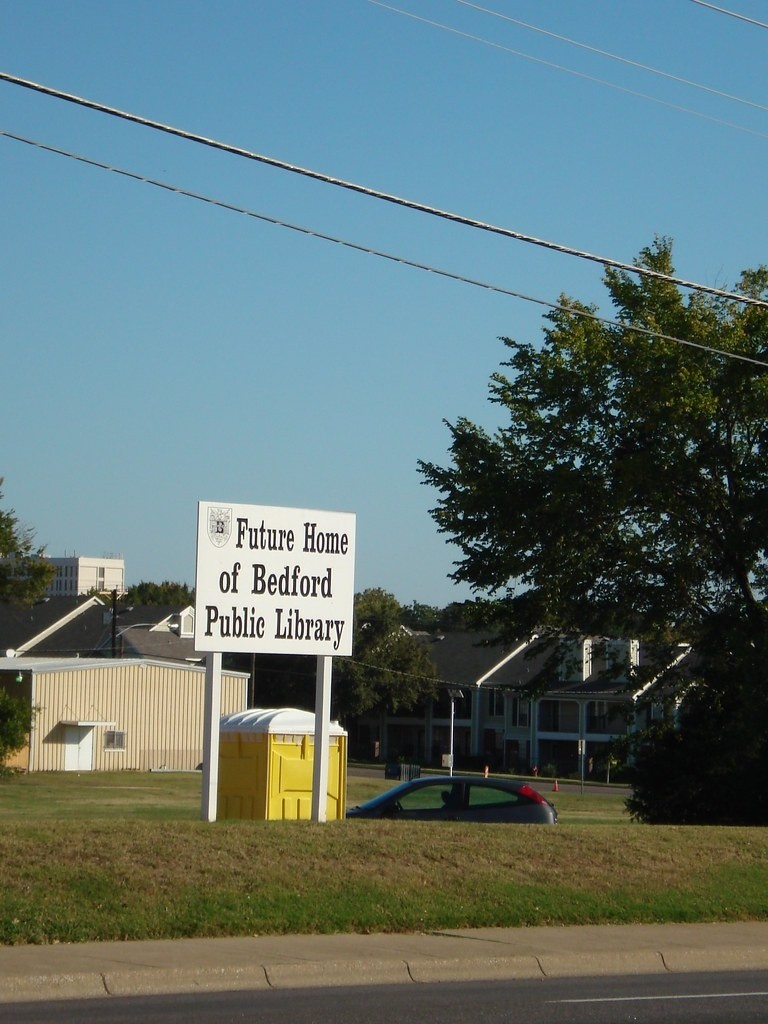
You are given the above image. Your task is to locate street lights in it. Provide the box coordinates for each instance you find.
[444,687,464,776]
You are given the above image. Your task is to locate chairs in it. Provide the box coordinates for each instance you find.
[440,790,450,807]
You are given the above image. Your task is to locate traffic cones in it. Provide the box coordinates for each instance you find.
[552,778,560,792]
[531,765,538,776]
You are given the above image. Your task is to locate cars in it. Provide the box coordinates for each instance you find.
[348,773,559,823]
